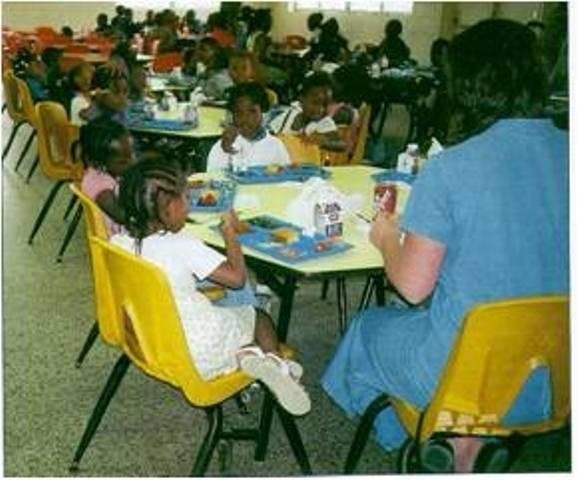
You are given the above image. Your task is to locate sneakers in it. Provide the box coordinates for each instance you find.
[427,440,455,474]
[474,443,507,472]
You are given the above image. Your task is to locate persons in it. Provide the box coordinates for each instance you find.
[526,21,567,99]
[207,83,292,310]
[375,19,411,69]
[107,157,312,416]
[318,15,569,473]
[417,37,450,145]
[10,4,366,166]
[71,119,134,236]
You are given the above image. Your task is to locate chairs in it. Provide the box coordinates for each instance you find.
[130,166,411,464]
[2,25,570,182]
[69,185,251,417]
[69,236,311,476]
[23,103,87,265]
[341,297,569,473]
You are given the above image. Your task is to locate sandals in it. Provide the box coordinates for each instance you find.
[235,345,303,382]
[240,353,313,416]
